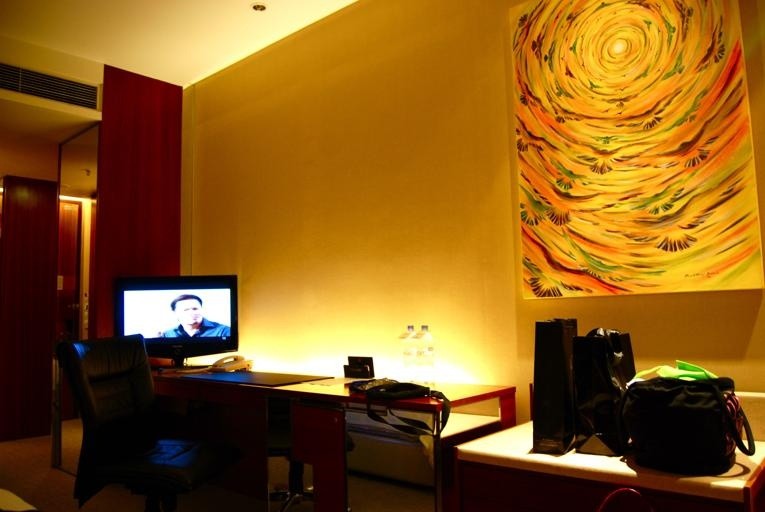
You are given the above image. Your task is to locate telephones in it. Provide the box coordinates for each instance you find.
[209,355,254,372]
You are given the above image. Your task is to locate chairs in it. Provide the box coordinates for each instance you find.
[55,334,241,510]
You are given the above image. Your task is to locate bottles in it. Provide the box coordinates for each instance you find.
[399,324,435,385]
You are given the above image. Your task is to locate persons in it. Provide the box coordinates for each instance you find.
[161,294,231,338]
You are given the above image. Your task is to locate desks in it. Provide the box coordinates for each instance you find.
[151,367,517,511]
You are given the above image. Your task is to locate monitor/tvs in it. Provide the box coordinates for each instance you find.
[108,275,239,369]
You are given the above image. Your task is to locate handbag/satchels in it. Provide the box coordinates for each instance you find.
[533,320,756,477]
[364,383,451,437]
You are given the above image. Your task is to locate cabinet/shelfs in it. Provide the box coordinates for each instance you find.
[453,419,764,512]
[343,414,502,511]
